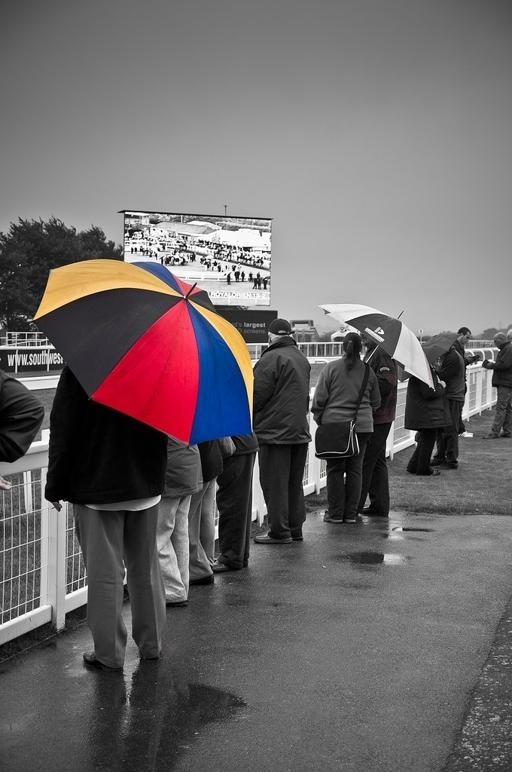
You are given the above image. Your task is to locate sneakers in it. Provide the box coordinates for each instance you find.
[460,431,474,438]
[345,517,358,522]
[483,436,501,439]
[324,515,343,523]
[500,433,511,436]
[432,468,442,474]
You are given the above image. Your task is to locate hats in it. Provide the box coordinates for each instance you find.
[268,319,292,335]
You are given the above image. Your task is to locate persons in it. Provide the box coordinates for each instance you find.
[188,440,223,585]
[44,366,167,670]
[152,429,201,607]
[440,326,482,469]
[360,330,395,517]
[211,436,259,571]
[434,346,465,469]
[252,319,311,541]
[483,332,512,440]
[310,332,381,524]
[1,369,45,462]
[405,353,451,476]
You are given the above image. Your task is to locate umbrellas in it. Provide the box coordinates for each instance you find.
[28,259,254,443]
[320,303,435,395]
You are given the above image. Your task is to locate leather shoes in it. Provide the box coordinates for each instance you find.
[439,462,458,468]
[192,575,216,585]
[431,459,440,464]
[210,560,233,571]
[165,599,188,607]
[289,529,302,540]
[359,506,390,516]
[255,534,293,544]
[146,645,162,661]
[84,651,113,670]
